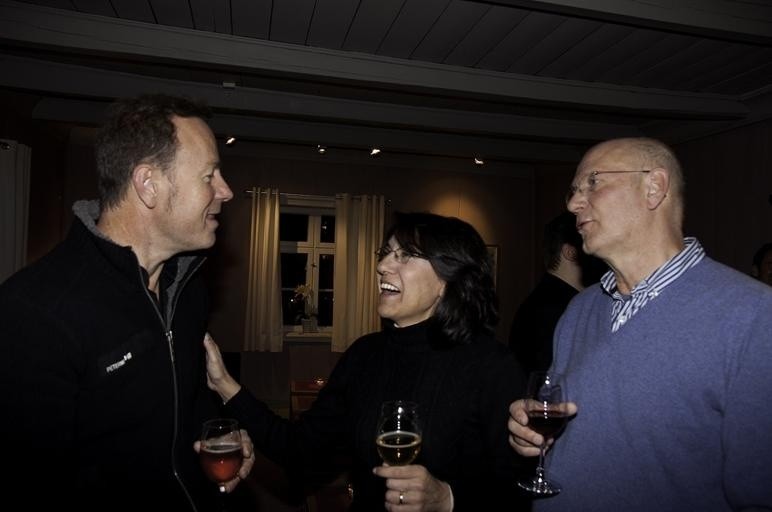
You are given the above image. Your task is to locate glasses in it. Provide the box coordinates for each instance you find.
[373,245,426,265]
[565,167,648,204]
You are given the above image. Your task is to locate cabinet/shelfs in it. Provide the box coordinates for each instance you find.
[287,378,327,421]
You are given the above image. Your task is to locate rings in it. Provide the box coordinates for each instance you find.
[398,492,404,506]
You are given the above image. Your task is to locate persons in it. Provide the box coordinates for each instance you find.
[202,210,534,512]
[507,135,772,512]
[510,210,608,399]
[751,242,772,286]
[0,93,257,512]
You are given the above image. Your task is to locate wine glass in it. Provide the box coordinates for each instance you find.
[517,371,568,496]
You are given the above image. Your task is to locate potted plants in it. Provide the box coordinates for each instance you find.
[292,283,321,336]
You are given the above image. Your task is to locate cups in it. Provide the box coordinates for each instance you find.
[376,399,423,468]
[199,417,243,484]
